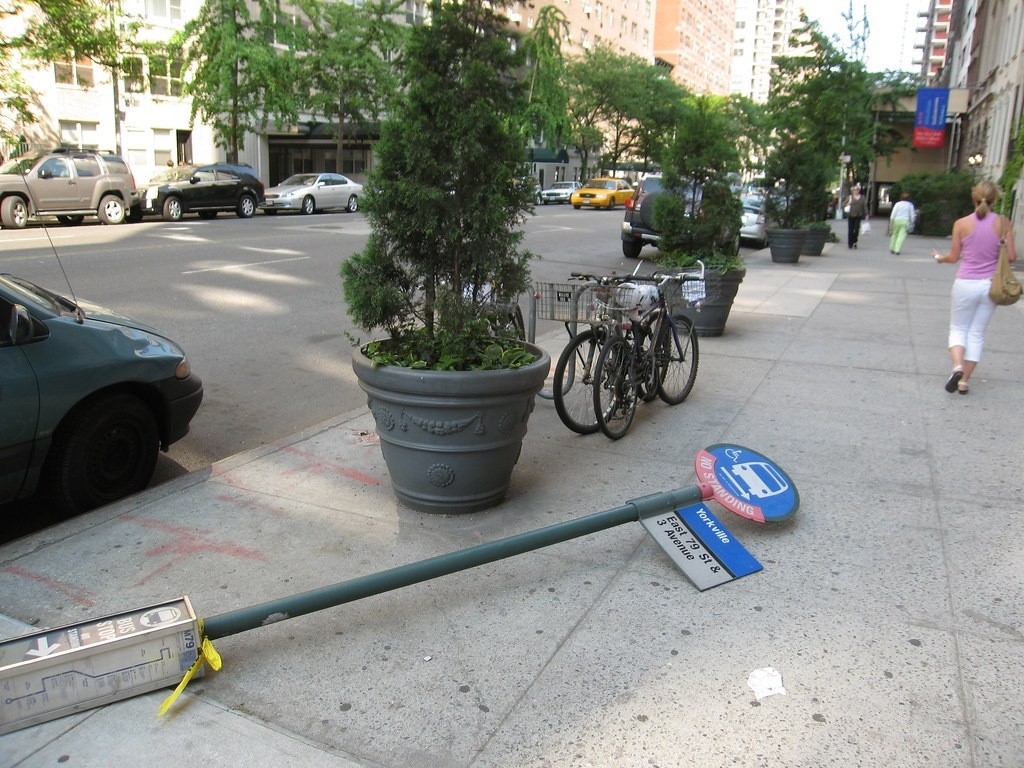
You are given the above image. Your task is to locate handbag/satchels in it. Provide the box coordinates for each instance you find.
[906,222,915,233]
[988,215,1024,306]
[844,195,852,213]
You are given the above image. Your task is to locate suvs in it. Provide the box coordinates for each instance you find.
[622,170,742,262]
[0,149,140,229]
[135,162,265,222]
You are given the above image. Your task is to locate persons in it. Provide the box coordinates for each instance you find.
[841,186,868,250]
[889,192,915,255]
[932,181,1016,395]
[165,159,179,180]
[178,159,186,167]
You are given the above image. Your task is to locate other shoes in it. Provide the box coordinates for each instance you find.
[957,382,969,395]
[944,365,964,393]
[854,244,857,249]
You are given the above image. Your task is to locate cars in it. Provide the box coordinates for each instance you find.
[572,176,636,210]
[518,176,542,206]
[0,273,203,523]
[721,183,837,250]
[258,172,364,215]
[541,180,584,206]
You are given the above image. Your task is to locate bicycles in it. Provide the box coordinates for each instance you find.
[535,256,706,440]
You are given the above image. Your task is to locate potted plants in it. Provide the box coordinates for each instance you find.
[338,0,553,516]
[754,130,833,263]
[649,93,755,337]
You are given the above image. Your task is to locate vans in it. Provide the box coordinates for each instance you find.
[877,183,895,216]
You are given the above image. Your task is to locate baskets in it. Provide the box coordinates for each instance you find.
[535,281,636,325]
[638,265,723,309]
